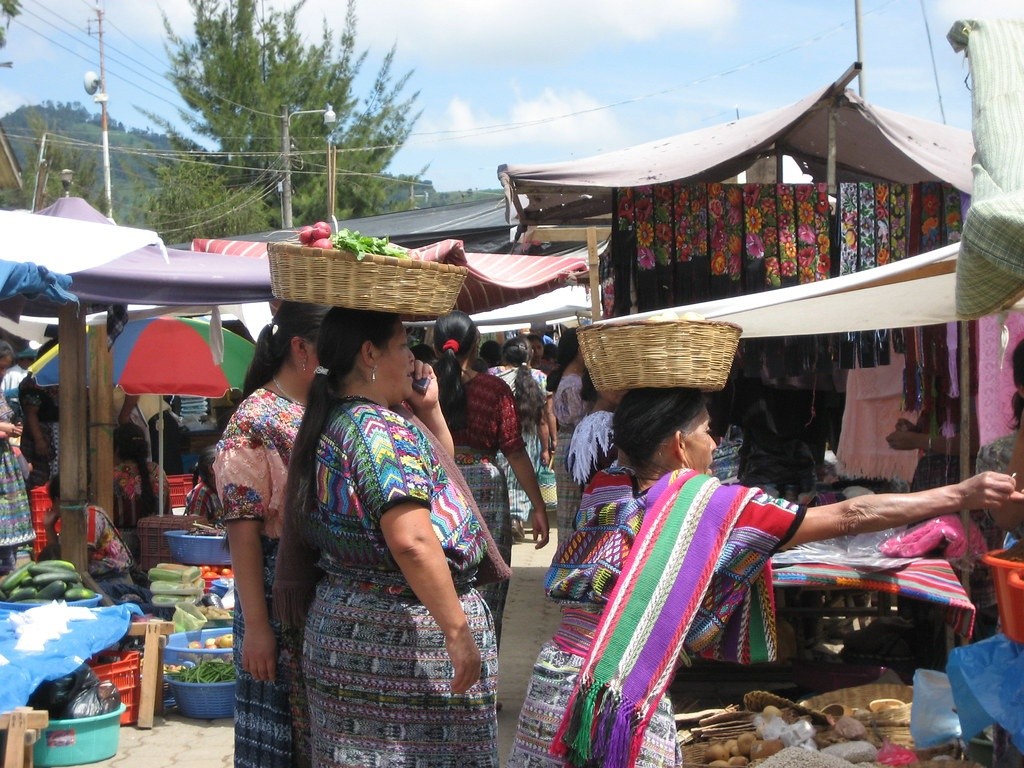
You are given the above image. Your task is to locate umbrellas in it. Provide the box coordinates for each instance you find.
[27,315,255,517]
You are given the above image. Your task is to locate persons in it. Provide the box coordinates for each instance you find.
[0,339,61,575]
[973,340,1024,542]
[566,366,620,532]
[269,308,510,768]
[545,327,593,549]
[36,470,136,586]
[885,322,996,646]
[184,442,227,529]
[213,299,330,768]
[507,385,1015,768]
[411,309,561,713]
[105,304,182,530]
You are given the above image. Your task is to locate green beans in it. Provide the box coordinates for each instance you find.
[163,660,238,684]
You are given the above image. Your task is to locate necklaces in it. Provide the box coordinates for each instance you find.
[344,394,380,405]
[272,375,305,405]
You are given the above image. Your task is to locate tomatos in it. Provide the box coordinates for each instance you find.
[198,564,230,577]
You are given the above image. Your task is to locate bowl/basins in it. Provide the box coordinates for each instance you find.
[980,548,1024,645]
[165,626,235,664]
[33,702,127,767]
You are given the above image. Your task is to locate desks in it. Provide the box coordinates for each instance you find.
[773,555,977,693]
[177,429,222,451]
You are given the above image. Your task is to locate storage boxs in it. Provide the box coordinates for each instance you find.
[30,481,61,565]
[91,649,142,725]
[165,472,196,508]
[177,452,199,472]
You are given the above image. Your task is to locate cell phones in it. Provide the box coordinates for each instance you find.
[411,374,430,395]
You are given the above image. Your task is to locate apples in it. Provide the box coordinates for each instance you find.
[187,633,233,650]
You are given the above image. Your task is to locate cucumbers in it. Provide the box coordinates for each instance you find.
[0,560,95,603]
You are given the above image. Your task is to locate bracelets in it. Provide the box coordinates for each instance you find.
[33,435,45,442]
[927,435,931,449]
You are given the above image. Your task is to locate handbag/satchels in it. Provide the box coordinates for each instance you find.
[537,451,558,511]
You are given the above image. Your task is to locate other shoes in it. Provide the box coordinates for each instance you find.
[511,517,525,543]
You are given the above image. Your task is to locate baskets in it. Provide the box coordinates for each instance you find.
[575,320,742,393]
[267,240,468,322]
